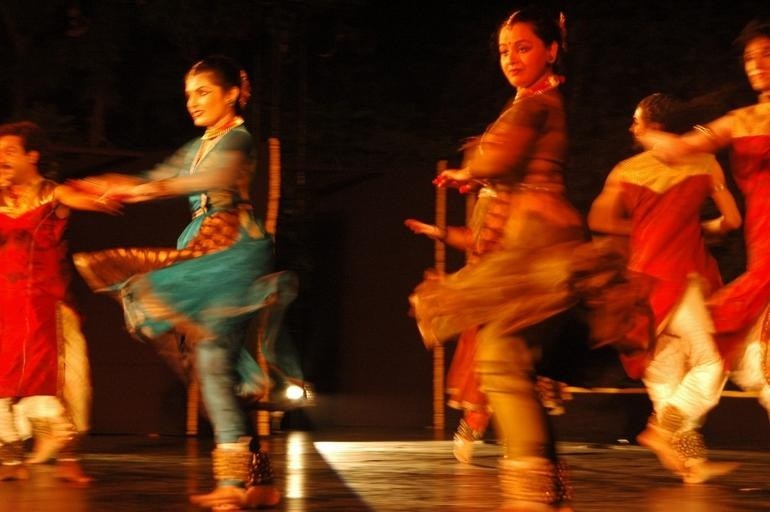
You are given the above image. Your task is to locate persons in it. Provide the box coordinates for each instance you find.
[622,18,769,427]
[400,129,510,465]
[2,118,129,486]
[432,3,592,503]
[574,90,748,486]
[54,54,309,510]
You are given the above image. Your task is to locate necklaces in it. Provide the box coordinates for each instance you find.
[199,115,244,144]
[512,74,563,102]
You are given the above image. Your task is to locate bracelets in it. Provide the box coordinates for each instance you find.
[435,223,449,244]
